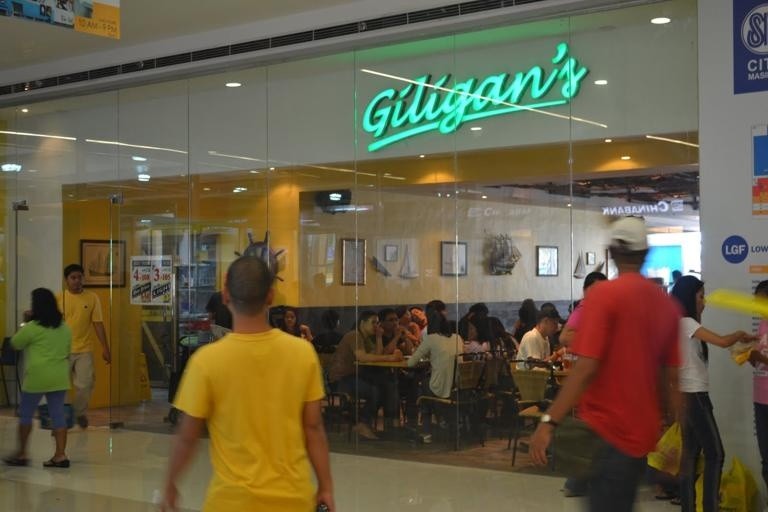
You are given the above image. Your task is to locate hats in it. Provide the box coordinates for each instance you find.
[537,307,565,324]
[609,217,648,251]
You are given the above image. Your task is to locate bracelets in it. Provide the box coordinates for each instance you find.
[18,322,26,328]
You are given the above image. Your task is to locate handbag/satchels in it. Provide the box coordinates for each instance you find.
[551,415,609,474]
[37,403,73,430]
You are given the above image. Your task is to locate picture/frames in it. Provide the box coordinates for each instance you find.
[80,239,126,288]
[440,241,467,275]
[536,245,558,276]
[586,252,596,264]
[342,238,366,284]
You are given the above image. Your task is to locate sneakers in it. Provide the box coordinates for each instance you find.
[77,415,89,428]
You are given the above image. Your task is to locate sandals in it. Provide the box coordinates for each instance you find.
[343,416,381,442]
[409,432,432,445]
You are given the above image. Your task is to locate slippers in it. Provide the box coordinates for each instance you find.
[655,490,682,505]
[4,454,70,468]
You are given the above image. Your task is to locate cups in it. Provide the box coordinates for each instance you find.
[561,352,577,370]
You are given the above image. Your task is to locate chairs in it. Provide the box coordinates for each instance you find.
[324,343,580,469]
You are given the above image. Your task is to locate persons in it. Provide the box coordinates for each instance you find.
[557,273,610,474]
[55,264,111,427]
[3,287,73,467]
[672,275,751,511]
[270,299,582,444]
[159,257,336,512]
[669,270,681,295]
[527,218,685,512]
[747,280,768,487]
[206,292,232,342]
